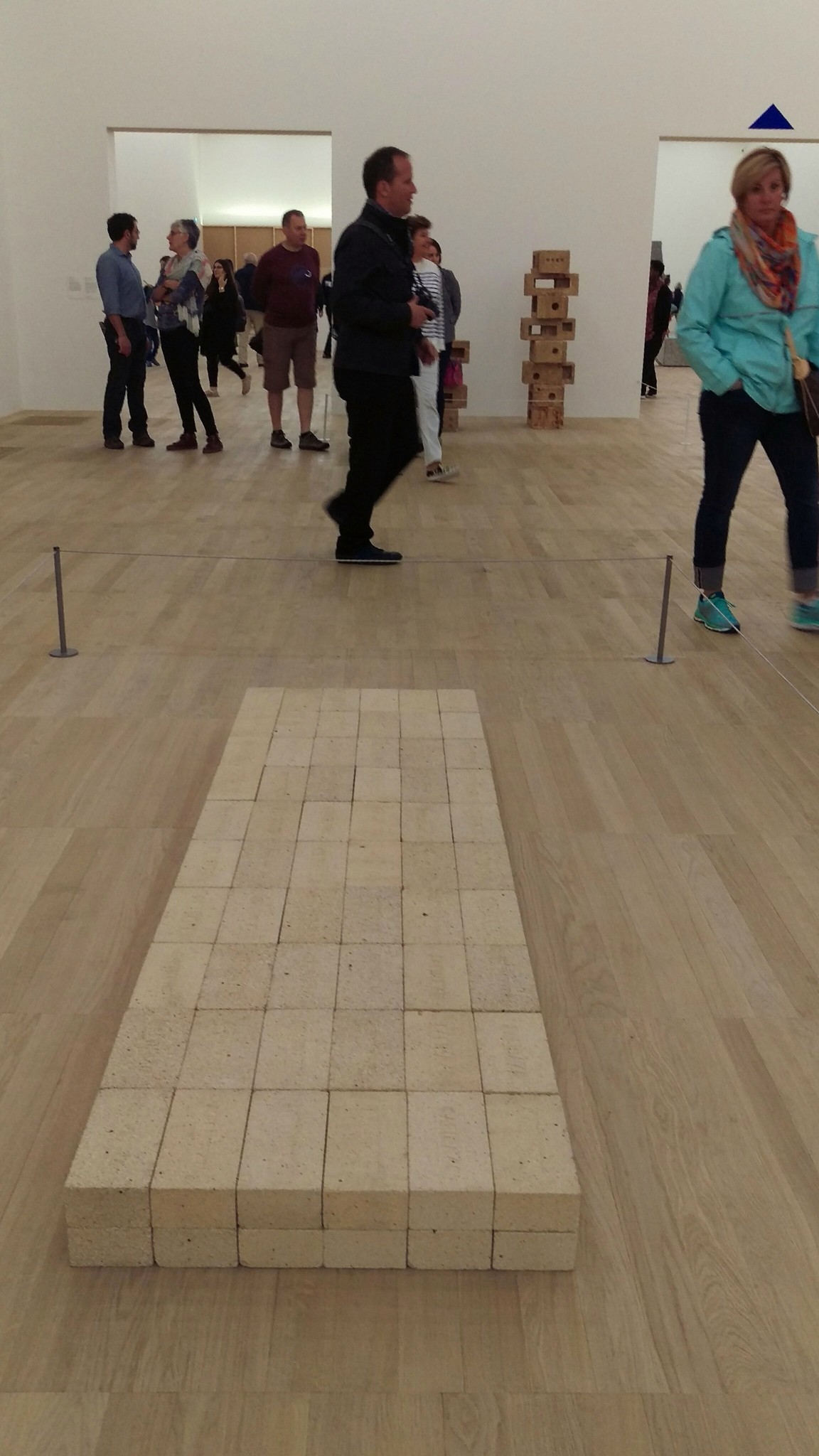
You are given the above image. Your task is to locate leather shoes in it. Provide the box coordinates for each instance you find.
[790,596,819,631]
[335,536,403,565]
[133,432,155,447]
[327,491,374,540]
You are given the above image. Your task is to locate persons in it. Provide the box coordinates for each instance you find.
[640,259,683,398]
[676,149,819,634]
[324,147,438,566]
[95,211,461,480]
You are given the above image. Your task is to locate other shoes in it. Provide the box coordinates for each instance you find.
[151,357,160,366]
[238,362,248,368]
[104,436,124,449]
[146,360,152,367]
[426,464,460,482]
[258,362,264,367]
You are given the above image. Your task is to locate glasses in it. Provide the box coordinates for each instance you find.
[170,231,186,236]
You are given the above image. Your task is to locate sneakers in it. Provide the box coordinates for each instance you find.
[203,435,223,453]
[298,431,330,451]
[270,429,292,448]
[204,387,219,397]
[693,591,741,633]
[242,372,251,395]
[167,432,198,450]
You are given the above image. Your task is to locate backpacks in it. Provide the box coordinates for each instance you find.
[231,294,247,333]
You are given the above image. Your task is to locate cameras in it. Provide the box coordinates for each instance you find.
[415,288,441,322]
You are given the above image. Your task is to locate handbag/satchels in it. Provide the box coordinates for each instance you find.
[792,357,819,437]
